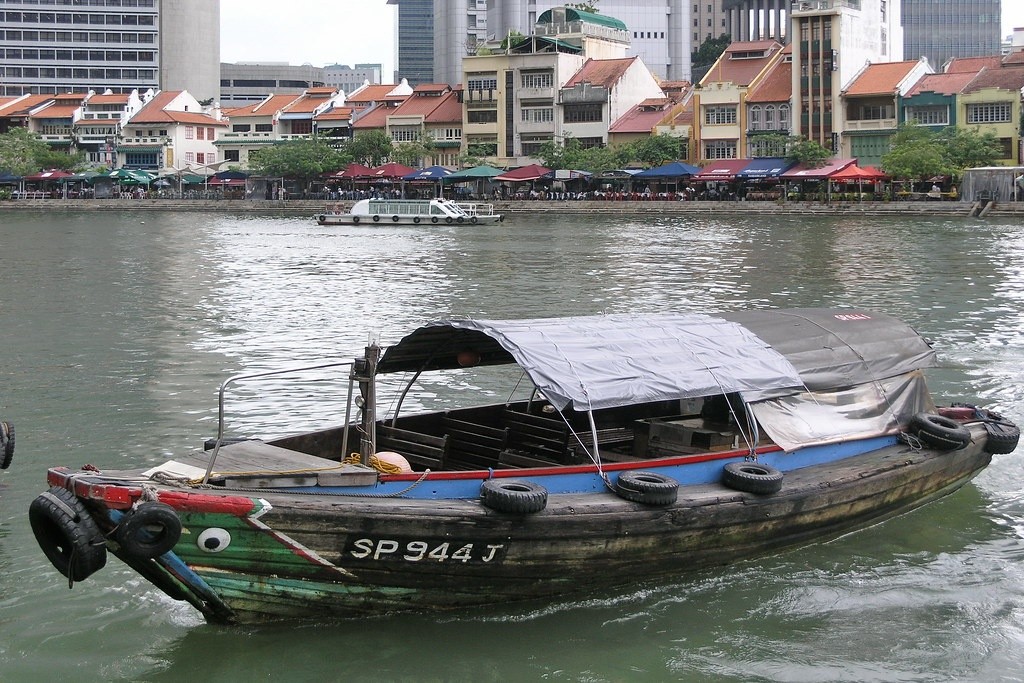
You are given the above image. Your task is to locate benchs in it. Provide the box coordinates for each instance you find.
[441,414,510,471]
[376,420,450,471]
[497,452,564,469]
[500,407,571,464]
[567,427,635,448]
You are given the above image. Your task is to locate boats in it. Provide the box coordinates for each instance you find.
[28,308,1020,624]
[314,199,505,225]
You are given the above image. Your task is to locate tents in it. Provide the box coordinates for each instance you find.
[442,166,506,198]
[631,162,700,192]
[0,169,156,198]
[828,165,893,201]
[319,163,418,199]
[402,166,455,198]
[182,170,251,184]
[493,164,552,192]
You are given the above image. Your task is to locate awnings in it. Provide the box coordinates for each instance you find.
[690,157,857,197]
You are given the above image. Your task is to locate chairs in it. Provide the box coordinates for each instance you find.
[66,190,229,200]
[510,191,736,201]
[306,190,375,201]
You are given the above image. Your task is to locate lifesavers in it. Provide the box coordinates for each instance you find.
[432,216,438,223]
[952,402,1020,454]
[617,470,680,505]
[29,485,107,582]
[353,216,359,222]
[471,216,477,223]
[392,215,399,221]
[373,215,379,221]
[320,215,326,221]
[724,461,783,493]
[481,476,548,514]
[414,217,420,223]
[446,215,452,222]
[118,501,182,557]
[910,412,971,450]
[457,216,464,222]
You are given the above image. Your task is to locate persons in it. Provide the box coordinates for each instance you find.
[709,187,716,194]
[686,186,695,191]
[608,185,612,192]
[645,185,651,193]
